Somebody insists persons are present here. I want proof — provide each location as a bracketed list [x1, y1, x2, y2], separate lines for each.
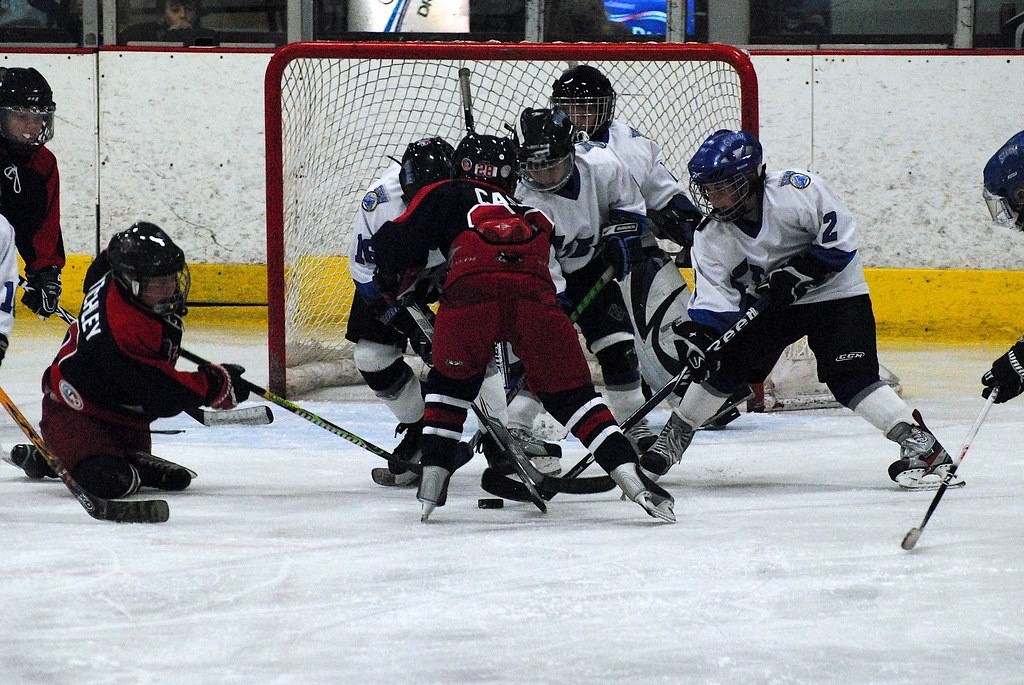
[0, 222, 252, 500]
[635, 129, 966, 490]
[0, 67, 66, 318]
[980, 131, 1024, 404]
[0, 0, 84, 44]
[117, 0, 219, 45]
[0, 214, 19, 367]
[346, 64, 739, 523]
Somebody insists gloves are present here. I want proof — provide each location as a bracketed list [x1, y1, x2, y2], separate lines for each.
[372, 260, 423, 307]
[0, 333, 9, 366]
[981, 341, 1024, 404]
[591, 206, 649, 282]
[21, 265, 62, 318]
[652, 193, 703, 245]
[555, 290, 574, 317]
[671, 320, 722, 384]
[738, 262, 816, 317]
[198, 361, 250, 410]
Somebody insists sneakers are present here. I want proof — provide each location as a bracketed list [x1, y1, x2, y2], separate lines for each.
[506, 428, 562, 478]
[388, 414, 426, 486]
[130, 449, 197, 493]
[639, 407, 700, 482]
[416, 442, 474, 521]
[0, 444, 60, 480]
[593, 431, 677, 523]
[697, 407, 741, 430]
[701, 383, 755, 427]
[624, 419, 659, 461]
[884, 408, 967, 492]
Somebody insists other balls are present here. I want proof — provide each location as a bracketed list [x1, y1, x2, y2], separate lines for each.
[478, 498, 504, 509]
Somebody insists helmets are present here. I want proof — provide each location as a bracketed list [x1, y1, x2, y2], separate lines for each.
[399, 137, 455, 205]
[451, 131, 520, 197]
[518, 107, 578, 194]
[983, 130, 1024, 213]
[552, 65, 614, 142]
[0, 66, 56, 155]
[687, 129, 762, 224]
[108, 220, 191, 318]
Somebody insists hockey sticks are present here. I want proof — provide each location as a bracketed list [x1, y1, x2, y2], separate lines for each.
[0, 386, 170, 521]
[466, 399, 549, 513]
[901, 386, 1000, 549]
[405, 300, 616, 493]
[17, 274, 273, 427]
[175, 342, 475, 476]
[468, 267, 614, 447]
[458, 67, 511, 388]
[480, 294, 772, 503]
